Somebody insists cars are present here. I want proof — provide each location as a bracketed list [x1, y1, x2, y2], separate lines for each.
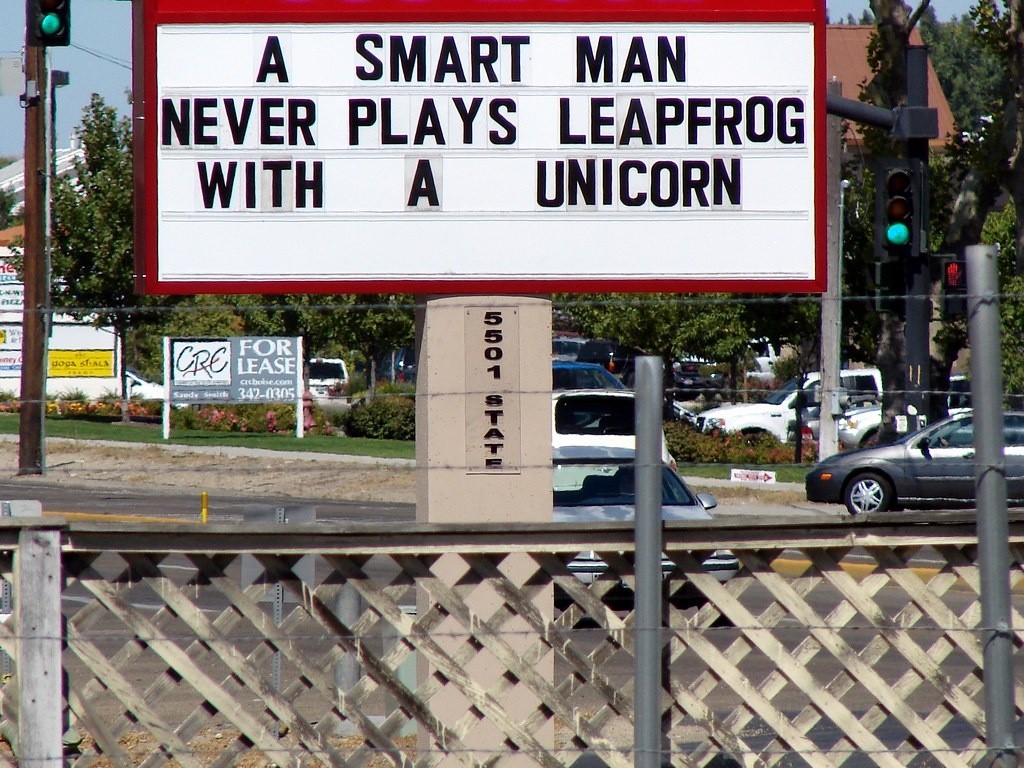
[550, 388, 679, 472]
[544, 445, 741, 594]
[671, 360, 724, 398]
[805, 407, 1024, 515]
[552, 356, 633, 392]
[123, 368, 190, 409]
[694, 369, 1009, 450]
[553, 336, 642, 382]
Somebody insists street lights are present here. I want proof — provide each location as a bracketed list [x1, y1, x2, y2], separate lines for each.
[50, 68, 71, 180]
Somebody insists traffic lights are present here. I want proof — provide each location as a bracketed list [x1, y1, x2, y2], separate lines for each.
[874, 161, 919, 259]
[941, 259, 967, 293]
[27, 0, 72, 47]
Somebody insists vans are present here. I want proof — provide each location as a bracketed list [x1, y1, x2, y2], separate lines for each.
[381, 346, 417, 384]
[306, 359, 352, 408]
